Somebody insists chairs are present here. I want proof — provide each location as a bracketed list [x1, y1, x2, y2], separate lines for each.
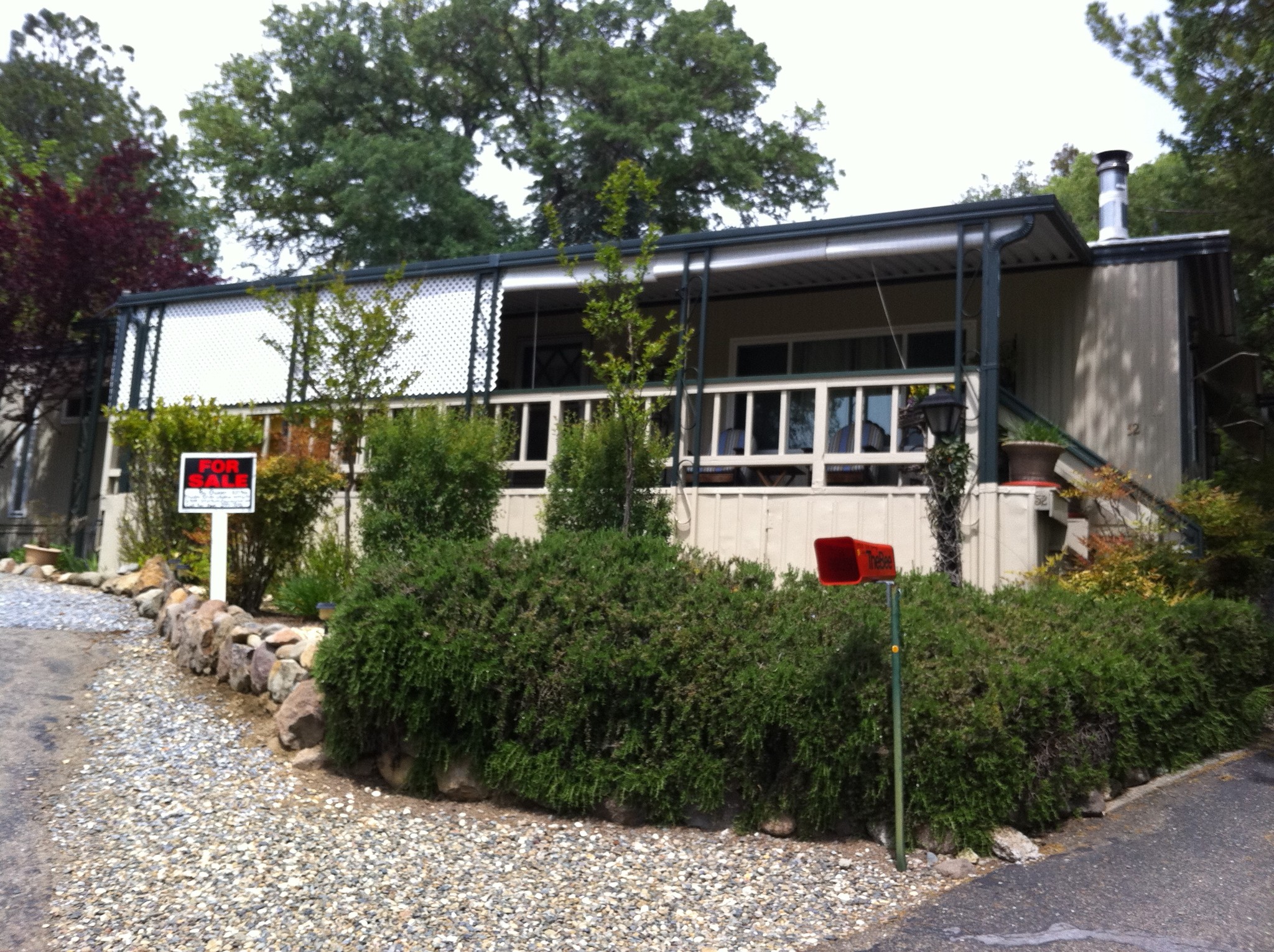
[800, 420, 890, 486]
[685, 428, 757, 487]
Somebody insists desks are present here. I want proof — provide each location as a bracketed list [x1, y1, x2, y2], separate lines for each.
[750, 462, 806, 487]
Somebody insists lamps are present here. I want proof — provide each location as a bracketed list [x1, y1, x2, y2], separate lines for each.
[919, 385, 965, 439]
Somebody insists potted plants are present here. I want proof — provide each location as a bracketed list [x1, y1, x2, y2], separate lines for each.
[22, 536, 63, 567]
[998, 414, 1070, 480]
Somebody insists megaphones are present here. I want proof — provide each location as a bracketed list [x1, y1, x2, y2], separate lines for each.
[813, 534, 896, 585]
